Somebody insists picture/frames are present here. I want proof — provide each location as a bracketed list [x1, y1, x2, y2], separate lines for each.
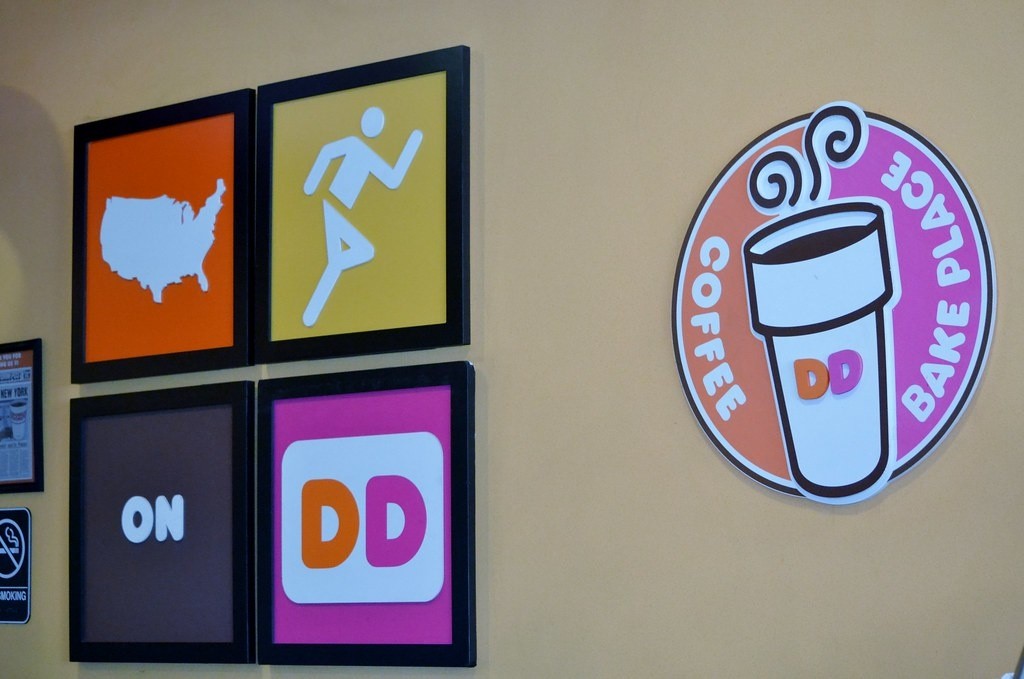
[70, 88, 251, 383]
[257, 361, 479, 667]
[69, 381, 252, 663]
[0, 338, 45, 493]
[254, 45, 470, 364]
